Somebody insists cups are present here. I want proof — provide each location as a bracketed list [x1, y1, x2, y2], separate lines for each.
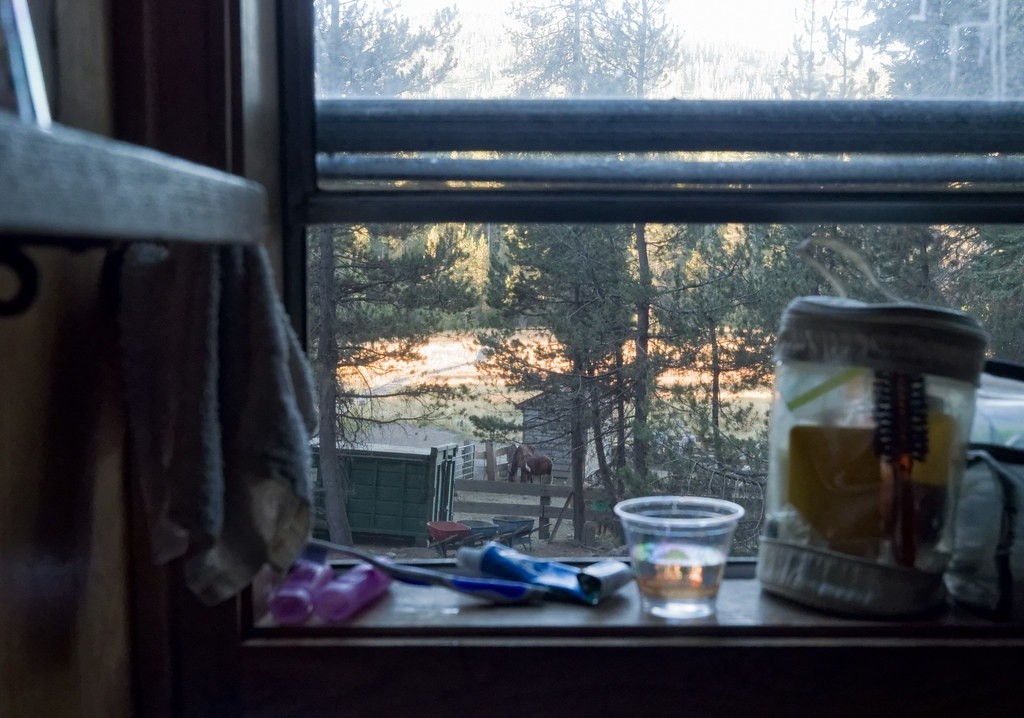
[613, 497, 747, 619]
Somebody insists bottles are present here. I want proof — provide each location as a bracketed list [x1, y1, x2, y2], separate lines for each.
[313, 554, 394, 622]
[269, 546, 334, 622]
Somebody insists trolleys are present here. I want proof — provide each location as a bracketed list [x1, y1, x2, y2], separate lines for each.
[426, 515, 552, 559]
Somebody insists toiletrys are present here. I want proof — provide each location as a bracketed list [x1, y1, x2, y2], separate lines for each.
[453, 539, 634, 603]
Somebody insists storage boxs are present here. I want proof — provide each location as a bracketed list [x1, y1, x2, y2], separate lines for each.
[311, 440, 459, 538]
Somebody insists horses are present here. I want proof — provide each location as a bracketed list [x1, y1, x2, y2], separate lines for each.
[509, 444, 553, 483]
